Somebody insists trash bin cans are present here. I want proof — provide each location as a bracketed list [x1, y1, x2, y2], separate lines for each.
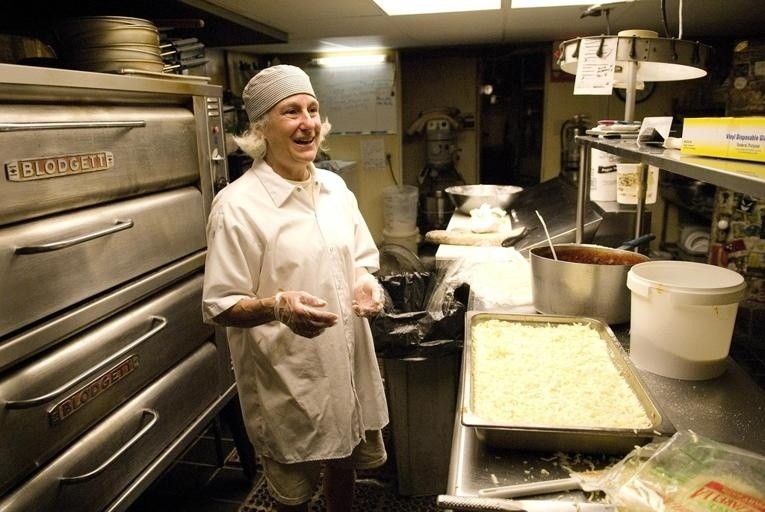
[371, 272, 470, 498]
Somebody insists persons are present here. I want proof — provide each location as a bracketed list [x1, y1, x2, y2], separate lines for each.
[202, 63, 391, 512]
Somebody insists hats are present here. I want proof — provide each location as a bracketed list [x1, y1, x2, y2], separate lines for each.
[243, 64, 316, 122]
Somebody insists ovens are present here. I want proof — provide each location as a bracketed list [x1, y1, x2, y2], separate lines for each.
[1, 66, 229, 512]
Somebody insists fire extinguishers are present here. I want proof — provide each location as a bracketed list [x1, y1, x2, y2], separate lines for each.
[561, 115, 588, 172]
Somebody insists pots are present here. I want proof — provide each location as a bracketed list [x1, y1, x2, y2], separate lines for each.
[74, 14, 210, 75]
[528, 230, 657, 326]
[661, 176, 716, 205]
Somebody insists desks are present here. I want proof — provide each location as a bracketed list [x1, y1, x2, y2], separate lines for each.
[436, 245, 765, 512]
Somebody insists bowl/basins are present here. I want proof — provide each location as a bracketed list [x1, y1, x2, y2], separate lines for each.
[443, 182, 524, 216]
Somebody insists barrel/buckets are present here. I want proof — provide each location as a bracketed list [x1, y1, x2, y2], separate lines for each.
[625, 260, 747, 381]
[382, 227, 419, 258]
[589, 148, 623, 202]
[616, 163, 659, 205]
